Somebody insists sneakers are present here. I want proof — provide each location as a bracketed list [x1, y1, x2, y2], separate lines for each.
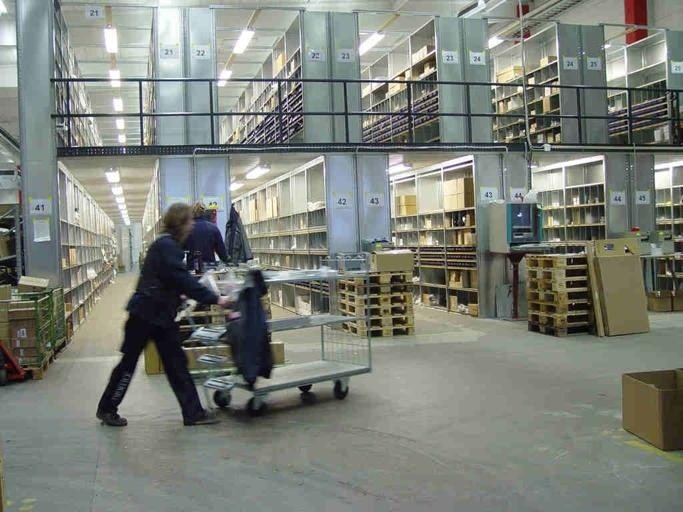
[94, 407, 128, 427]
[181, 410, 219, 426]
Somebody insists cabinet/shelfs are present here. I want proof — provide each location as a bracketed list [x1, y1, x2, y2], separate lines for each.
[0, 1, 681, 376]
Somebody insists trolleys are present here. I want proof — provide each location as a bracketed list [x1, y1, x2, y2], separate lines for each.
[179, 248, 377, 419]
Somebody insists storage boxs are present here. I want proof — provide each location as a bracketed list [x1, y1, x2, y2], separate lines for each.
[620, 365, 682, 454]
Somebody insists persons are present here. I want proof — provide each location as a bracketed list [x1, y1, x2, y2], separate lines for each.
[181, 202, 231, 268]
[96, 204, 235, 426]
